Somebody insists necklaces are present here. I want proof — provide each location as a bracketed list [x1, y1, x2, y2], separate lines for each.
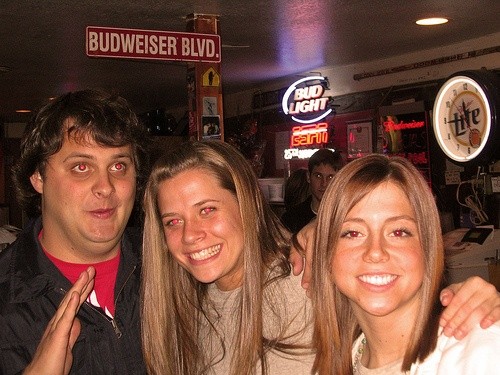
[348, 336, 368, 375]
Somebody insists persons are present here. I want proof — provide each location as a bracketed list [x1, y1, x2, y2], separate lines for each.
[0, 86, 321, 375]
[308, 152, 500, 375]
[282, 168, 312, 210]
[278, 148, 344, 233]
[135, 137, 499, 375]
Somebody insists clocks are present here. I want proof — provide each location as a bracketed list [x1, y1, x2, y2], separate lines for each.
[433, 70, 500, 167]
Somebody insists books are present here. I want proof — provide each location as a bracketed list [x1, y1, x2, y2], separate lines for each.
[462, 225, 494, 246]
